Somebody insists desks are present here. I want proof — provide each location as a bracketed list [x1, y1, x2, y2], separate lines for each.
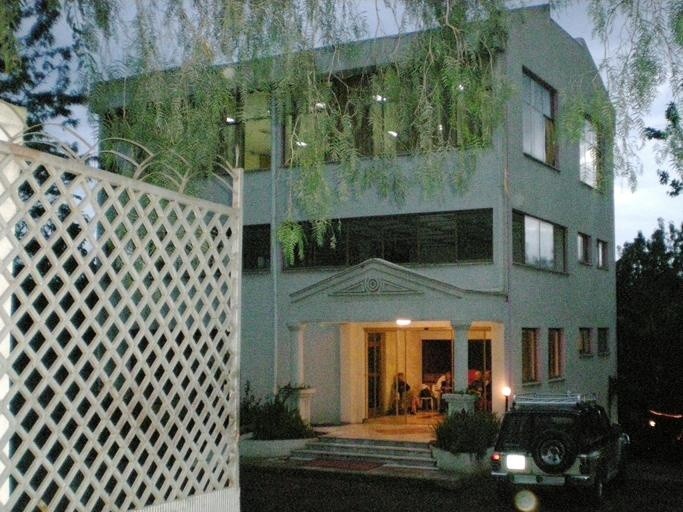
[421, 397, 433, 410]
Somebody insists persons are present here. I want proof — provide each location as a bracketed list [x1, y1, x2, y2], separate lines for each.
[437, 372, 452, 414]
[393, 373, 419, 416]
[485, 368, 490, 403]
[469, 368, 485, 409]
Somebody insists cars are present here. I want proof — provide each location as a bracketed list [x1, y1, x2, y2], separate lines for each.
[636, 398, 683, 458]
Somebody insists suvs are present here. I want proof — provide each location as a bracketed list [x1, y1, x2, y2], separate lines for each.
[490, 392, 632, 506]
